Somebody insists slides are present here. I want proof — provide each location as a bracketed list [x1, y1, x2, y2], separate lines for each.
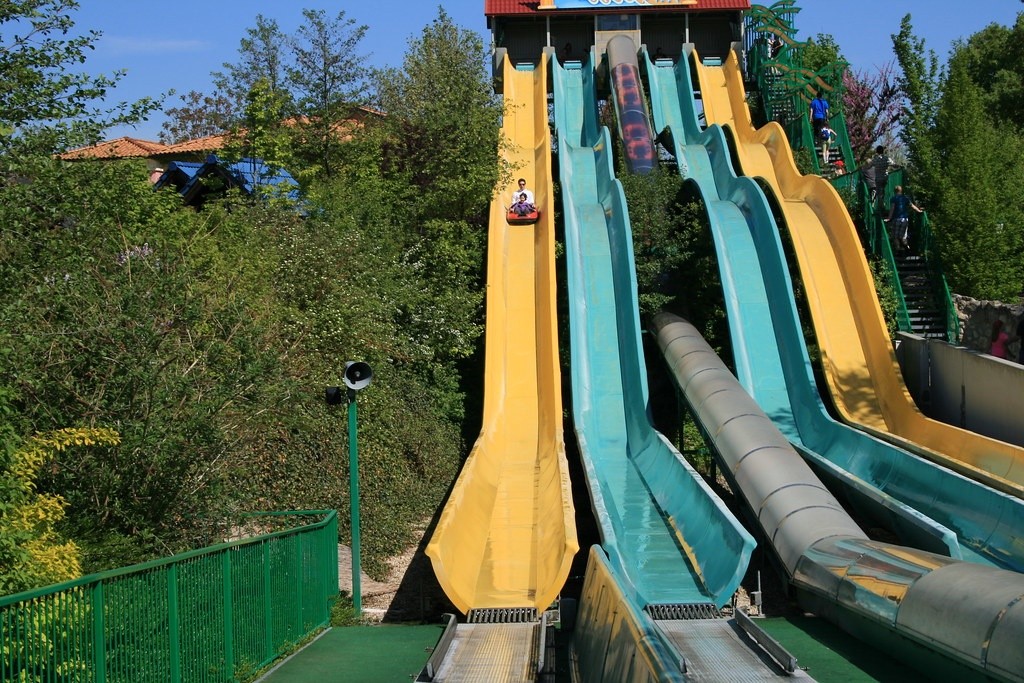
[424, 44, 1022, 614]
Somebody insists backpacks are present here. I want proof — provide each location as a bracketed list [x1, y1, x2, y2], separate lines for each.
[821, 128, 831, 141]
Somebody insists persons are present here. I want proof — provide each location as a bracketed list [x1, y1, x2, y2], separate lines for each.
[767, 34, 782, 74]
[985, 320, 1016, 360]
[509, 179, 536, 217]
[810, 92, 829, 147]
[814, 161, 846, 178]
[650, 48, 665, 64]
[862, 146, 889, 215]
[883, 185, 923, 250]
[1016, 312, 1024, 365]
[820, 123, 837, 165]
